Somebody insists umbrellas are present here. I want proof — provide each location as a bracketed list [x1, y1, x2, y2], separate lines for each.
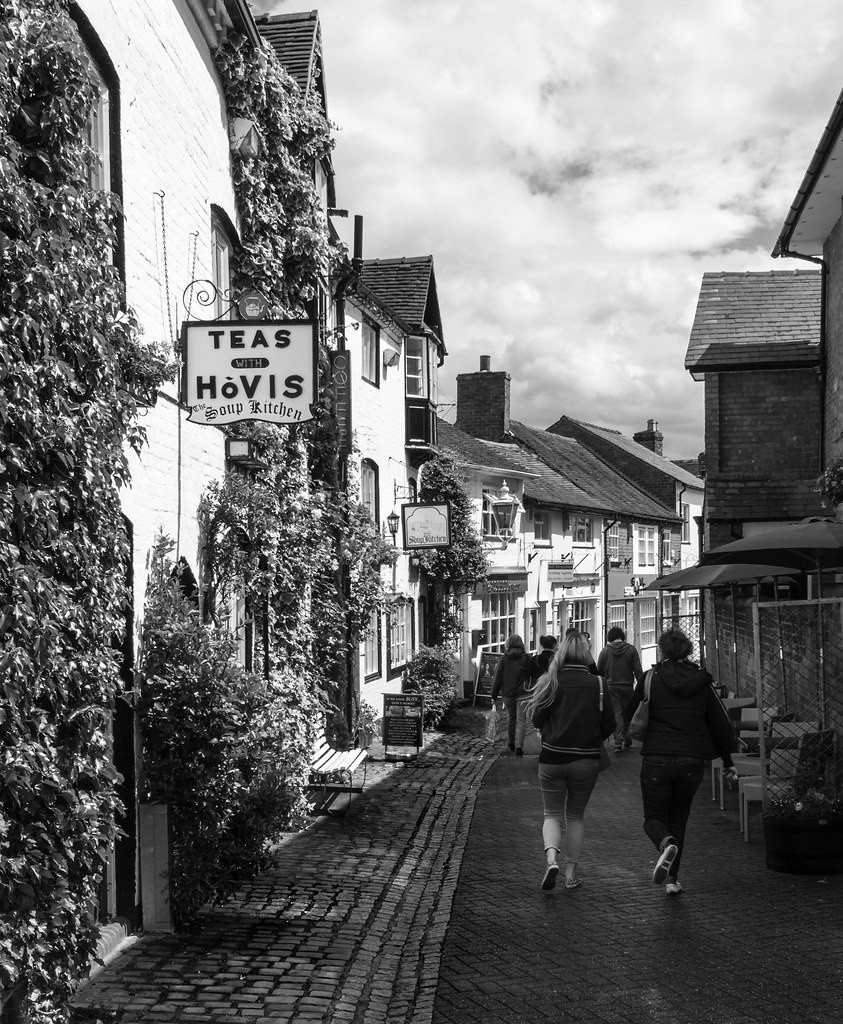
[635, 522, 843, 730]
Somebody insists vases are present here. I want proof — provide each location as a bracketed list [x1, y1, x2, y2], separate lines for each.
[833, 503, 843, 520]
[760, 812, 842, 875]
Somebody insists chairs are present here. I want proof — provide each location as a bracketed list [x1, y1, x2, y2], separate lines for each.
[737, 722, 818, 832]
[710, 707, 778, 802]
[743, 729, 836, 841]
[719, 712, 794, 809]
[721, 696, 755, 721]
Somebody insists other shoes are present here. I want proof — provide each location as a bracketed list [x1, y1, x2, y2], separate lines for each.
[565, 873, 582, 889]
[614, 747, 621, 752]
[516, 748, 523, 755]
[653, 845, 678, 884]
[508, 743, 515, 751]
[665, 881, 681, 894]
[541, 860, 559, 890]
[624, 742, 632, 748]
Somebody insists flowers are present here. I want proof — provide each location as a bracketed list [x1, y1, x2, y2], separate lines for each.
[816, 456, 843, 509]
[763, 776, 842, 817]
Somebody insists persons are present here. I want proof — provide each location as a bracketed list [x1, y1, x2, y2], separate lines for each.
[625, 630, 738, 895]
[531, 627, 643, 753]
[491, 635, 530, 754]
[521, 633, 617, 889]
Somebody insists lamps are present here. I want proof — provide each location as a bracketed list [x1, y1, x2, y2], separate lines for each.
[386, 510, 400, 534]
[480, 478, 520, 551]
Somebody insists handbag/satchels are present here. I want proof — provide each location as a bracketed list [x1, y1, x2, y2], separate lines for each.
[599, 740, 610, 772]
[628, 666, 655, 742]
[485, 704, 500, 743]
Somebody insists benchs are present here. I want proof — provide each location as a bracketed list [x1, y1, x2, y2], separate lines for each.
[303, 711, 367, 815]
